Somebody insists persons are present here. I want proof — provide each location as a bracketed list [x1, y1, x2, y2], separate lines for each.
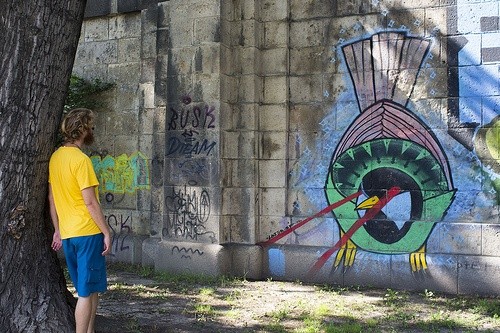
[48, 108, 113, 333]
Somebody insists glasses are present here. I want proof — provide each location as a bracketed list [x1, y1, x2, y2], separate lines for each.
[87, 124, 97, 131]
